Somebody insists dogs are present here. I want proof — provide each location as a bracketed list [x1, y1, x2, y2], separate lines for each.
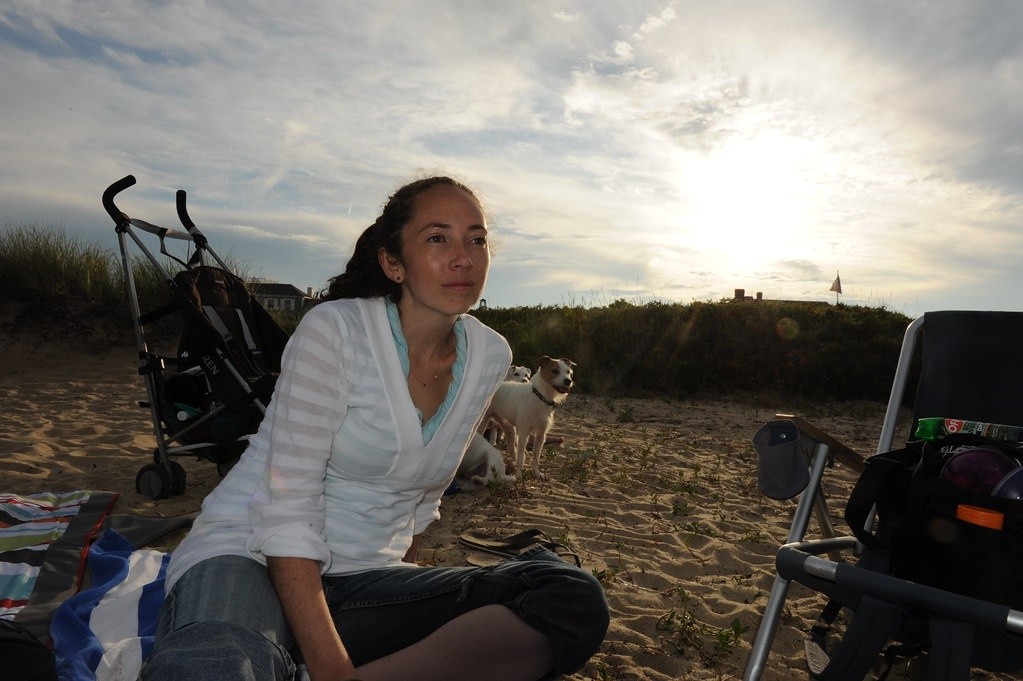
[476, 356, 578, 480]
[486, 364, 531, 445]
[453, 430, 516, 486]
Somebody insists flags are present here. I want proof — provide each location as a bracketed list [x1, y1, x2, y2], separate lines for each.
[829, 276, 843, 294]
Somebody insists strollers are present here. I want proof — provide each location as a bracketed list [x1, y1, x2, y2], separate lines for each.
[103, 175, 296, 498]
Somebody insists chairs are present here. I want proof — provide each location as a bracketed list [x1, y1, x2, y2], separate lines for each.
[730, 309, 1023, 681]
[175, 257, 291, 446]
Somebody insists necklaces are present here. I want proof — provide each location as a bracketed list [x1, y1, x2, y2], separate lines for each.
[406, 331, 456, 388]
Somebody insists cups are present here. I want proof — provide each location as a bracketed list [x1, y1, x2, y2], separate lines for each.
[956, 504, 1005, 530]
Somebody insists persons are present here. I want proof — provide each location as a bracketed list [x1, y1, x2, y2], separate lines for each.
[124, 176, 611, 681]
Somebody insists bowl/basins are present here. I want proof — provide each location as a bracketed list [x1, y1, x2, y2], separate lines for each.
[941, 445, 1017, 496]
[991, 466, 1023, 501]
